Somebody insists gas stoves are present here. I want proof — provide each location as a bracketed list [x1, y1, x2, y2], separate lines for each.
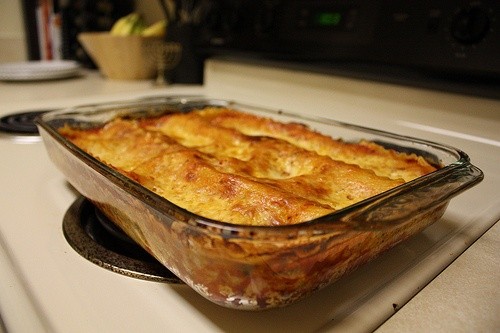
[1, 84, 500, 333]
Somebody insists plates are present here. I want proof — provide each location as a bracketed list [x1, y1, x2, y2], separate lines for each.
[0, 61, 81, 80]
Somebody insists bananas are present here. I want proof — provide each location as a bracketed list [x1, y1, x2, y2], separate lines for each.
[110, 11, 167, 35]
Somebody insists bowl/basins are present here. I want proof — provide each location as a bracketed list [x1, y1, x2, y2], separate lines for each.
[79, 32, 165, 80]
[34, 95, 485, 311]
